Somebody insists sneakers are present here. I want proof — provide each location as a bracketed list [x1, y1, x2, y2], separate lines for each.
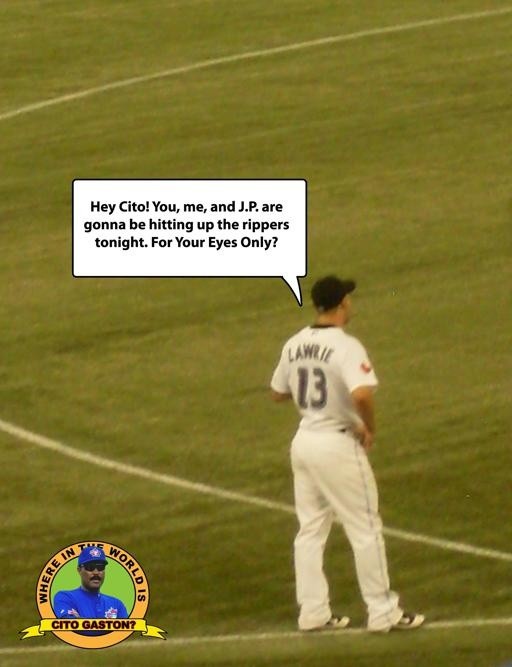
[299, 616, 349, 632]
[374, 612, 425, 632]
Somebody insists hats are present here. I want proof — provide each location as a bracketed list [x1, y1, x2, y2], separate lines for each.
[311, 278, 355, 302]
[78, 547, 108, 567]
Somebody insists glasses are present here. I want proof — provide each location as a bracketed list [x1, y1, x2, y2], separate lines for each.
[79, 564, 106, 571]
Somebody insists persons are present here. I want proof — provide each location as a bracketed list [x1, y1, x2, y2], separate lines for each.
[54, 547, 129, 636]
[270, 275, 426, 633]
